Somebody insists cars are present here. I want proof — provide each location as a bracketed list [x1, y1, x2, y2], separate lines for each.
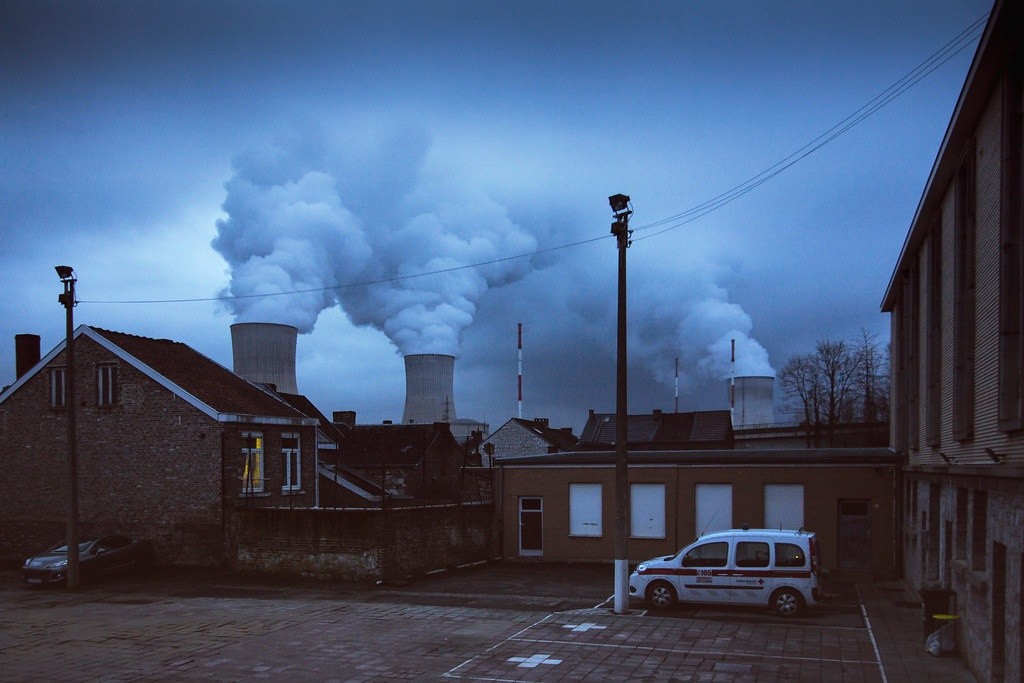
[629, 529, 824, 617]
[22, 533, 154, 584]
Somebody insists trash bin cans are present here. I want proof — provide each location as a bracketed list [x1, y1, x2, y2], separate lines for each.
[915, 577, 958, 645]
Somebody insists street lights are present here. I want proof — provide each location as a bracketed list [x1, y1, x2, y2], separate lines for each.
[609, 195, 632, 614]
[56, 266, 80, 588]
